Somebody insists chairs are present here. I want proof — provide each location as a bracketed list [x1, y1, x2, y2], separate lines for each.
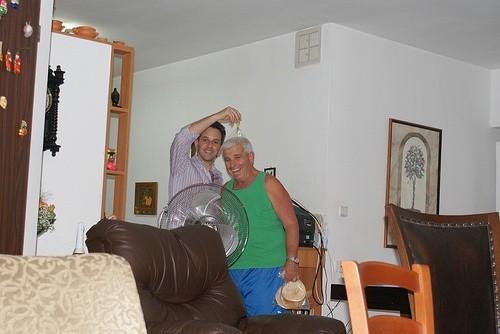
[385, 204, 500, 334]
[342, 259, 435, 334]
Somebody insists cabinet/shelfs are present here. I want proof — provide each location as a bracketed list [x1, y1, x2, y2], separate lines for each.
[101, 45, 134, 222]
[331, 284, 412, 319]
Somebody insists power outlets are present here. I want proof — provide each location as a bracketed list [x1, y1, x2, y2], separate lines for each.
[339, 206, 348, 217]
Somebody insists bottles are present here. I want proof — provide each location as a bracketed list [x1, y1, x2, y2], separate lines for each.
[72, 221, 85, 254]
[111, 88, 119, 106]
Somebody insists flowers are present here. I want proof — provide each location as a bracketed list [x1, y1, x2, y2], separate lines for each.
[37, 198, 57, 238]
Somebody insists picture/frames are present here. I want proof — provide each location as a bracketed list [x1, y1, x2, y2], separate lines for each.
[384, 118, 442, 248]
[264, 168, 276, 177]
[134, 182, 158, 215]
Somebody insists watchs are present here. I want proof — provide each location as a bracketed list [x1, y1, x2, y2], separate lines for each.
[288, 256, 300, 264]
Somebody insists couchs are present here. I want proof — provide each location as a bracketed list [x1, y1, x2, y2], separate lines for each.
[0, 254, 148, 334]
[85, 217, 346, 334]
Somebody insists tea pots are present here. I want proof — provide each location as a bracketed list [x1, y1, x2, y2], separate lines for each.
[52, 20, 65, 32]
[72, 26, 98, 39]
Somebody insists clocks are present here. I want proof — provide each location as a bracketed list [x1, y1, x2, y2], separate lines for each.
[42, 63, 66, 156]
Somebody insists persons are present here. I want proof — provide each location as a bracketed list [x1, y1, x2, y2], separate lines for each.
[167, 107, 243, 229]
[220, 135, 302, 316]
